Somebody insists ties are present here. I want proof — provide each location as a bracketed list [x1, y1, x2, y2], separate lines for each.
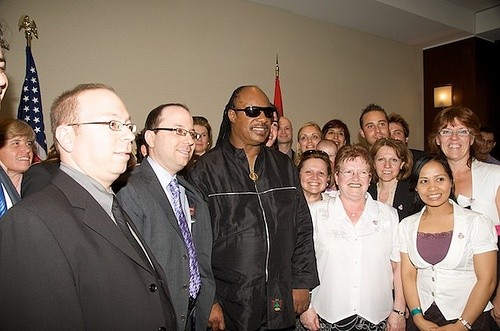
[170, 179, 201, 299]
[0, 184, 7, 217]
[112, 193, 178, 330]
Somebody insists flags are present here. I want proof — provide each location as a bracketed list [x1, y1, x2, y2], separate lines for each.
[17, 46, 47, 165]
[273, 79, 283, 117]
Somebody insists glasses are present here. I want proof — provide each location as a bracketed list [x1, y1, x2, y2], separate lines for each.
[301, 149, 328, 157]
[341, 168, 369, 178]
[439, 129, 469, 135]
[233, 106, 275, 118]
[151, 129, 198, 138]
[70, 120, 137, 131]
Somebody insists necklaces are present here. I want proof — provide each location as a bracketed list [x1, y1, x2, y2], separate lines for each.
[344, 207, 364, 217]
[451, 169, 470, 182]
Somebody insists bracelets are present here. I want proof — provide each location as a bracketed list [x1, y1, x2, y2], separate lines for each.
[457, 316, 472, 331]
[411, 307, 422, 317]
[393, 309, 407, 317]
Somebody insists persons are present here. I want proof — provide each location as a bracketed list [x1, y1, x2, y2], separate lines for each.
[359, 102, 390, 151]
[426, 105, 500, 328]
[116, 103, 217, 330]
[134, 128, 148, 165]
[316, 138, 339, 191]
[184, 85, 320, 331]
[387, 112, 426, 173]
[322, 119, 350, 153]
[1, 118, 37, 198]
[296, 151, 332, 203]
[185, 116, 213, 172]
[266, 103, 280, 148]
[0, 17, 21, 219]
[293, 122, 325, 167]
[471, 127, 500, 166]
[398, 153, 499, 331]
[296, 143, 408, 331]
[273, 115, 296, 163]
[367, 137, 421, 223]
[1, 83, 178, 331]
